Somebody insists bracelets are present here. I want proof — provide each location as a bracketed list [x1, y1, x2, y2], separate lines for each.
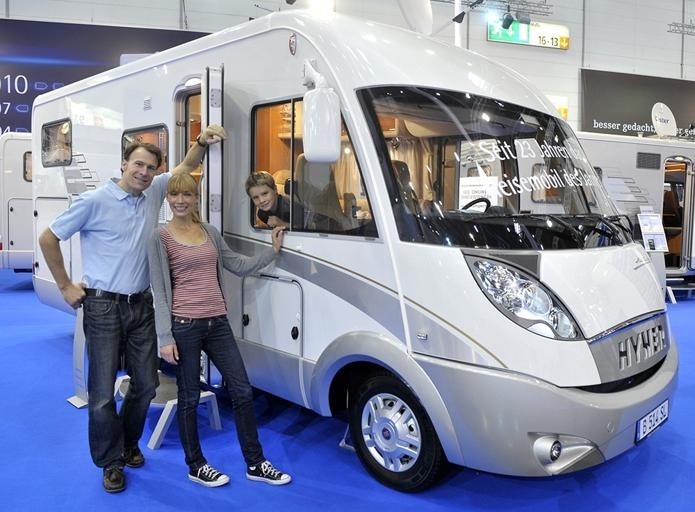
[196, 135, 205, 147]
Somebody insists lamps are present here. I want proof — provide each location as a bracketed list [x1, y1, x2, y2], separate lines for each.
[500, 4, 513, 30]
[515, 10, 531, 25]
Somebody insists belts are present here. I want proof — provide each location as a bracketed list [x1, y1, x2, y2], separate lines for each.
[82, 287, 143, 305]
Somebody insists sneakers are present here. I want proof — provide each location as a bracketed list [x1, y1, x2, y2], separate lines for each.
[188, 463, 230, 488]
[245, 459, 292, 486]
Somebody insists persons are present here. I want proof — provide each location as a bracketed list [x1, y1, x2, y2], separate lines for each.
[39, 125, 229, 494]
[244, 170, 341, 231]
[146, 173, 293, 486]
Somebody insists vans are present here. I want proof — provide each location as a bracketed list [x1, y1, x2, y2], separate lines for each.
[0, 132, 35, 271]
[455, 132, 695, 291]
[32, 9, 680, 493]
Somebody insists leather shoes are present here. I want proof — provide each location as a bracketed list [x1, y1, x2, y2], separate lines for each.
[120, 440, 145, 468]
[102, 463, 127, 493]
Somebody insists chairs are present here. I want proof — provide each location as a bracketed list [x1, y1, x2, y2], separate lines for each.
[271, 169, 300, 200]
[289, 154, 361, 235]
[663, 190, 683, 237]
[393, 159, 422, 216]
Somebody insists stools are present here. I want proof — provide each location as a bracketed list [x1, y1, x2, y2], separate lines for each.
[113, 368, 223, 450]
